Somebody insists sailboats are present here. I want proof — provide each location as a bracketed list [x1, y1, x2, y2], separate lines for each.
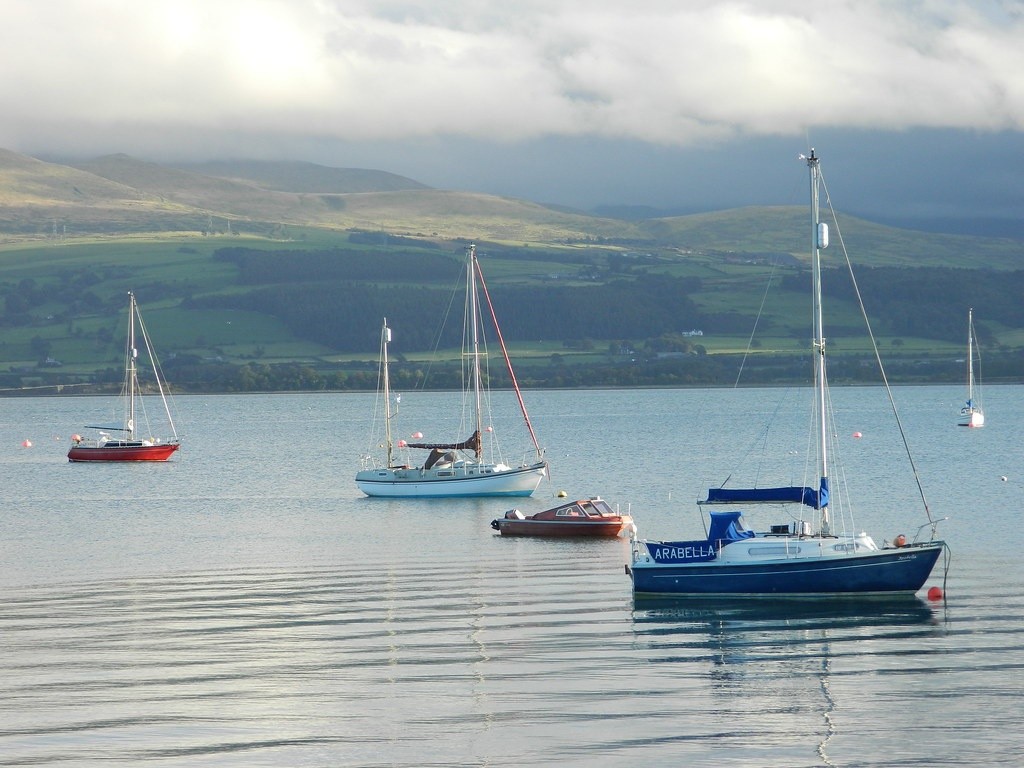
[622, 147, 956, 604]
[66, 290, 183, 462]
[958, 310, 987, 426]
[355, 244, 552, 499]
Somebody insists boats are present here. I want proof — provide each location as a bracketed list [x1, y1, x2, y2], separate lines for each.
[489, 496, 635, 539]
